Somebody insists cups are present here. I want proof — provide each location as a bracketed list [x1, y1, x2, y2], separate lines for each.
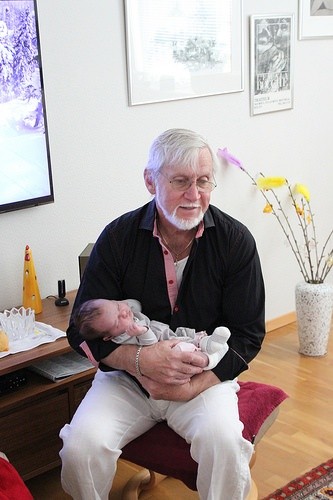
[0, 306, 36, 343]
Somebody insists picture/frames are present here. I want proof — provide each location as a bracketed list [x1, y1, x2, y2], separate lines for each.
[297, 0, 333, 40]
[122, 0, 244, 107]
[250, 12, 295, 117]
[0, 0, 55, 215]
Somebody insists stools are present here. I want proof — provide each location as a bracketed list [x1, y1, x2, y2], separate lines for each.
[118, 378, 290, 500]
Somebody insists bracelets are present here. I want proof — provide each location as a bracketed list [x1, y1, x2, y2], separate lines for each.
[136, 346, 143, 376]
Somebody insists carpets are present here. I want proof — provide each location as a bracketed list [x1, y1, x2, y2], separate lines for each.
[261, 457, 333, 500]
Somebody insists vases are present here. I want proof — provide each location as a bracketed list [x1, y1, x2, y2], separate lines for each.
[295, 279, 333, 358]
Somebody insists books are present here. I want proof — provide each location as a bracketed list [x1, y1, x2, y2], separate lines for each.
[29, 350, 96, 382]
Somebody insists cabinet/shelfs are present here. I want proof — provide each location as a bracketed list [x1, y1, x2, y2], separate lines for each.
[0, 288, 98, 483]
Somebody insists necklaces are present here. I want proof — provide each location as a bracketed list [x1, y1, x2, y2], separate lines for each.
[156, 215, 198, 265]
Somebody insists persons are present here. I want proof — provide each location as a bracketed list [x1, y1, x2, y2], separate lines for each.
[73, 299, 231, 370]
[59, 128, 266, 500]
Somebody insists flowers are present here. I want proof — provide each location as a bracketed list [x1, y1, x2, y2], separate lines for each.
[218, 148, 333, 285]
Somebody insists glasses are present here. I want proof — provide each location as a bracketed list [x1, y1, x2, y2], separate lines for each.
[156, 169, 217, 193]
